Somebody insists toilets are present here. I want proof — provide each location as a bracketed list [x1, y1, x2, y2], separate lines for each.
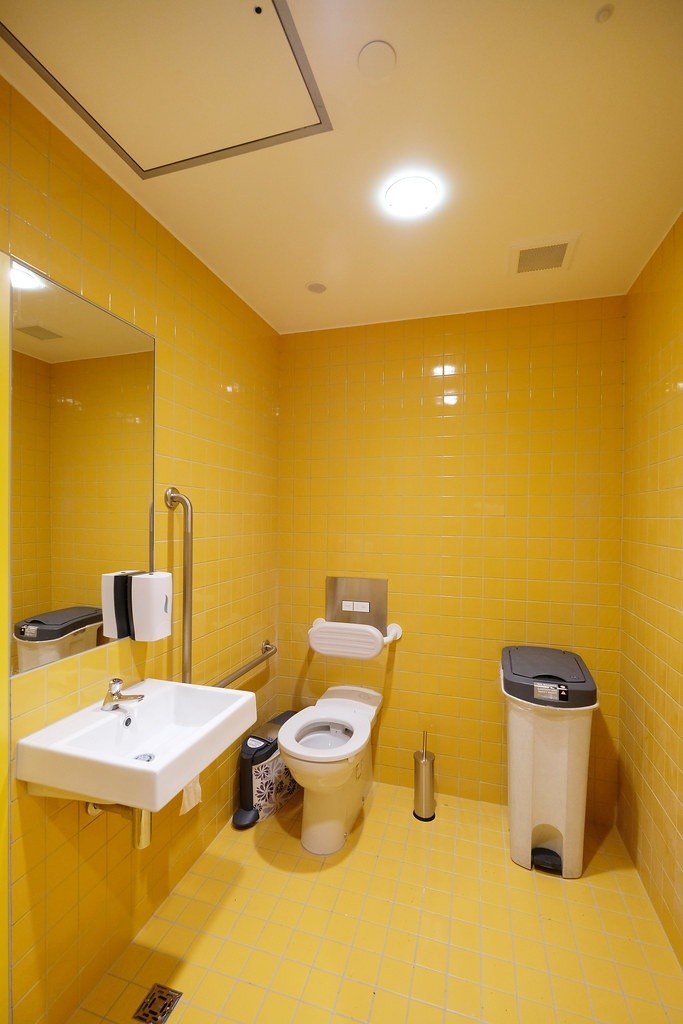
[276, 685, 383, 855]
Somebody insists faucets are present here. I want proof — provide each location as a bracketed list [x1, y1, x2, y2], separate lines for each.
[100, 678, 145, 711]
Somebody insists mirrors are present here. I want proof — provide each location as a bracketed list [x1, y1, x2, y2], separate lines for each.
[1, 250, 156, 677]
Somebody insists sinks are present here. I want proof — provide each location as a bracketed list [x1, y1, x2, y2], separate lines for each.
[16, 677, 257, 812]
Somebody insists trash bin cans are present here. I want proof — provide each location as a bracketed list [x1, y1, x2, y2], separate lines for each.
[13, 605, 103, 671]
[498, 643, 602, 882]
